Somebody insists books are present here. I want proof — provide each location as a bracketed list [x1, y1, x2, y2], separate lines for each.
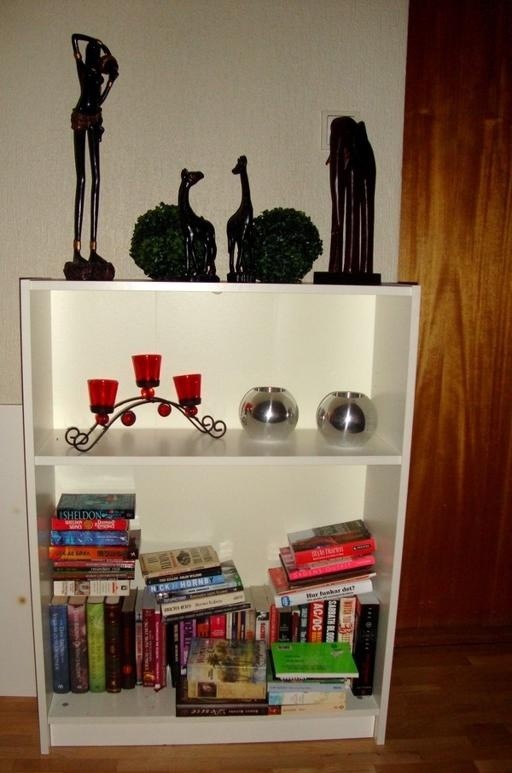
[46, 491, 383, 719]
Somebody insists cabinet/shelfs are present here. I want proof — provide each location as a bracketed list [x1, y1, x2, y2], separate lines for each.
[19, 278, 421, 755]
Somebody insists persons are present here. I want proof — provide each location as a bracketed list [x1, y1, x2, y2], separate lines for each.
[68, 30, 120, 270]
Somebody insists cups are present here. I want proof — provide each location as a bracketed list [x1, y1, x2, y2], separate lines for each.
[132, 355, 163, 386]
[172, 373, 203, 405]
[88, 377, 117, 414]
